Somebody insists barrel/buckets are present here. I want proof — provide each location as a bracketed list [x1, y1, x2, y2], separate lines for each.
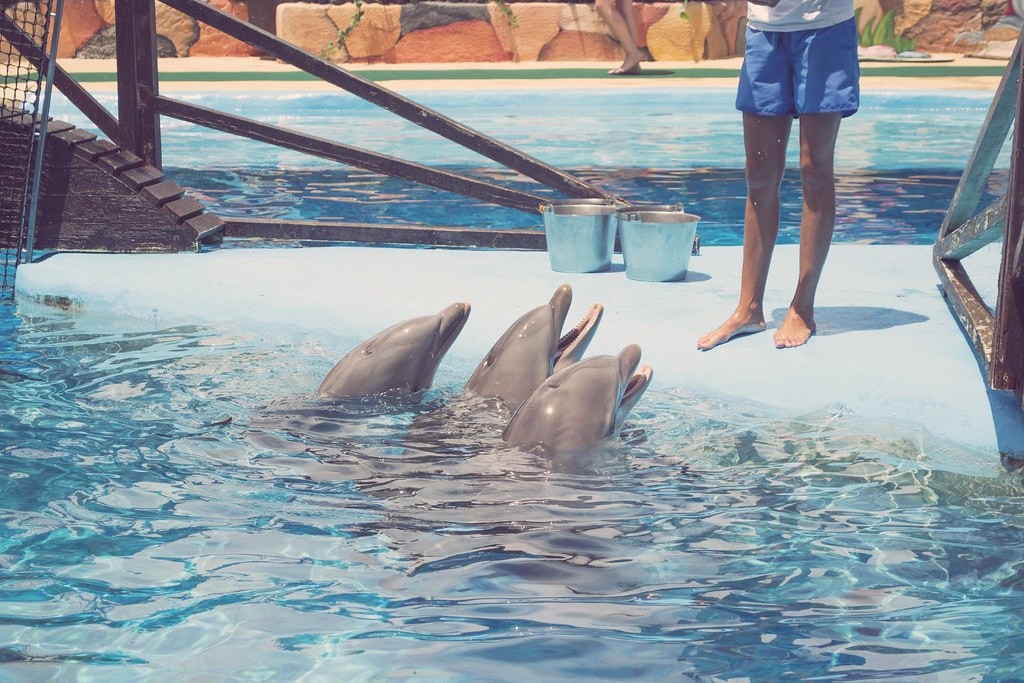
[614, 211, 701, 282]
[614, 204, 685, 215]
[542, 203, 618, 275]
[538, 197, 617, 214]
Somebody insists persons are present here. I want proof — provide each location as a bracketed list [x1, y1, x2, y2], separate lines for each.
[596, 0, 643, 75]
[697, 1, 862, 351]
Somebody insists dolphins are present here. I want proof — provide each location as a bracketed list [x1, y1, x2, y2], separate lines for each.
[462, 284, 604, 417]
[320, 304, 472, 406]
[504, 345, 654, 452]
